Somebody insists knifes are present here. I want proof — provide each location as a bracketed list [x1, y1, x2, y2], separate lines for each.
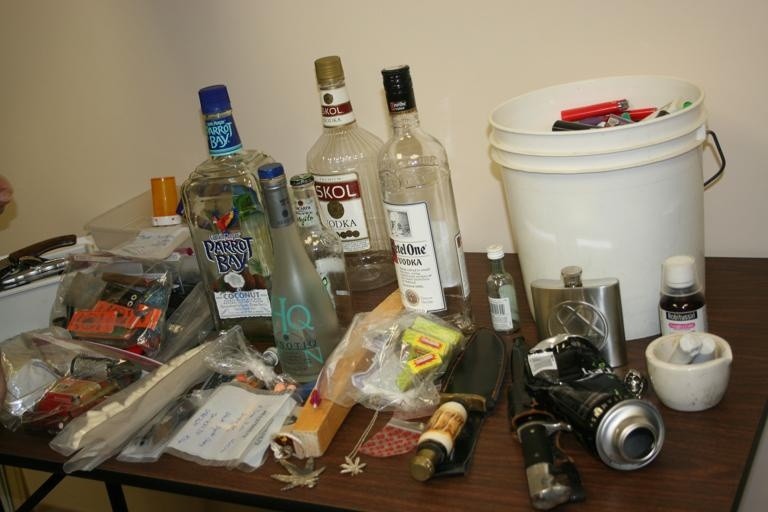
[507, 335, 573, 511]
[0, 233, 78, 273]
[409, 326, 506, 482]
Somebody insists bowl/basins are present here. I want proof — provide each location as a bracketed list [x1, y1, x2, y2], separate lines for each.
[645, 331, 733, 412]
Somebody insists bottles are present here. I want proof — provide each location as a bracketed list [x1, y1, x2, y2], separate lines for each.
[374, 64, 473, 325]
[528, 264, 627, 371]
[257, 162, 344, 417]
[288, 173, 357, 338]
[485, 242, 520, 336]
[68, 355, 131, 379]
[655, 256, 709, 336]
[304, 56, 397, 292]
[180, 83, 278, 348]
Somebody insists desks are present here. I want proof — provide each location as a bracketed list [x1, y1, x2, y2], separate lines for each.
[2, 217, 767, 512]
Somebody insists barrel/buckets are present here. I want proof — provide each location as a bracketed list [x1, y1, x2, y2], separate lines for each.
[488, 73, 726, 341]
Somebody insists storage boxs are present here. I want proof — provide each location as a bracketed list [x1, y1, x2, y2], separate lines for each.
[84, 184, 206, 289]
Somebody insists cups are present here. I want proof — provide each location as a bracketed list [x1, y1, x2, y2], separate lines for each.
[148, 176, 181, 227]
